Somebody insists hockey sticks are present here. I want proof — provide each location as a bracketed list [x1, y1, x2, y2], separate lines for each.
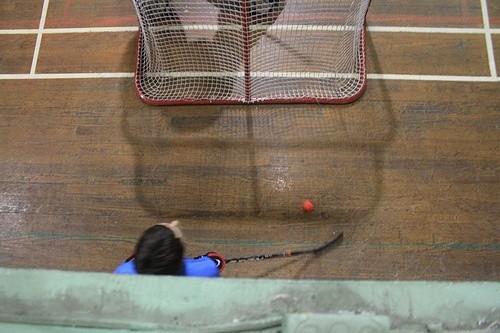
[225, 232, 345, 265]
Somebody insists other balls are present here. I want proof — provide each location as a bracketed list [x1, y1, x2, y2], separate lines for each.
[303, 199, 315, 211]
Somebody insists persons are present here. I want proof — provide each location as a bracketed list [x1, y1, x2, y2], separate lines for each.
[109, 219, 222, 279]
